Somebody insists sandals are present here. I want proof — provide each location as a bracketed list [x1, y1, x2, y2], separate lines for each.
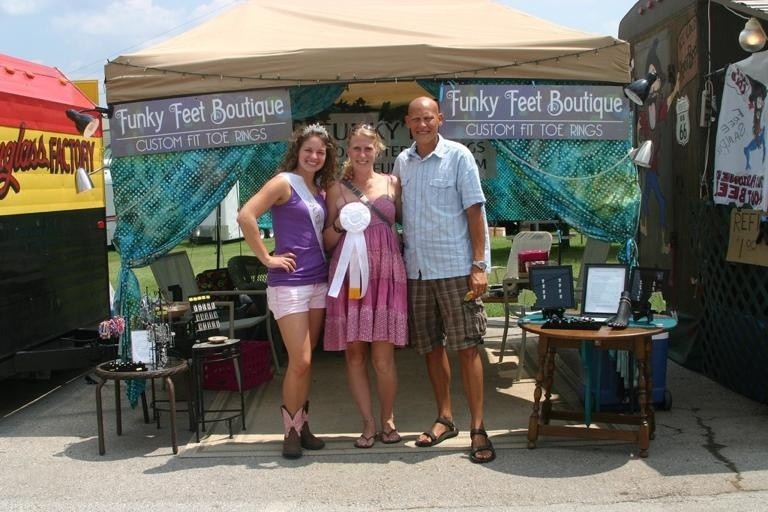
[470, 429, 496, 464]
[415, 418, 458, 448]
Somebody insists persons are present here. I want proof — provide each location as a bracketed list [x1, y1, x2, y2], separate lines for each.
[607, 290, 632, 329]
[389, 96, 497, 463]
[236, 120, 341, 460]
[323, 123, 403, 451]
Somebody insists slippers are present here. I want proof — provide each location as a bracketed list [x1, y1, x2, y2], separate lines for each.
[353, 434, 377, 449]
[381, 429, 402, 445]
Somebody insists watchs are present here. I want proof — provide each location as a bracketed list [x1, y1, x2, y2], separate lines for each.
[471, 259, 489, 272]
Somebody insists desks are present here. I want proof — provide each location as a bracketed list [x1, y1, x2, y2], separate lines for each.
[95, 354, 189, 457]
[191, 339, 247, 444]
[517, 313, 681, 458]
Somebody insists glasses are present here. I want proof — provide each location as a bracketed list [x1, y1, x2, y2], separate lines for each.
[348, 123, 378, 143]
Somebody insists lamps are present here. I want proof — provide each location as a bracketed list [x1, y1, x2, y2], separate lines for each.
[624, 73, 660, 108]
[64, 105, 115, 138]
[735, 17, 767, 53]
[633, 139, 661, 168]
[73, 163, 114, 194]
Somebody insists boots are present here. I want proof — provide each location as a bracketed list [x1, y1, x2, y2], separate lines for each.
[300, 400, 325, 451]
[280, 404, 305, 459]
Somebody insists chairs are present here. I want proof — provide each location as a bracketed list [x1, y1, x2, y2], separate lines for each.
[229, 255, 301, 368]
[481, 231, 556, 362]
[149, 251, 281, 394]
